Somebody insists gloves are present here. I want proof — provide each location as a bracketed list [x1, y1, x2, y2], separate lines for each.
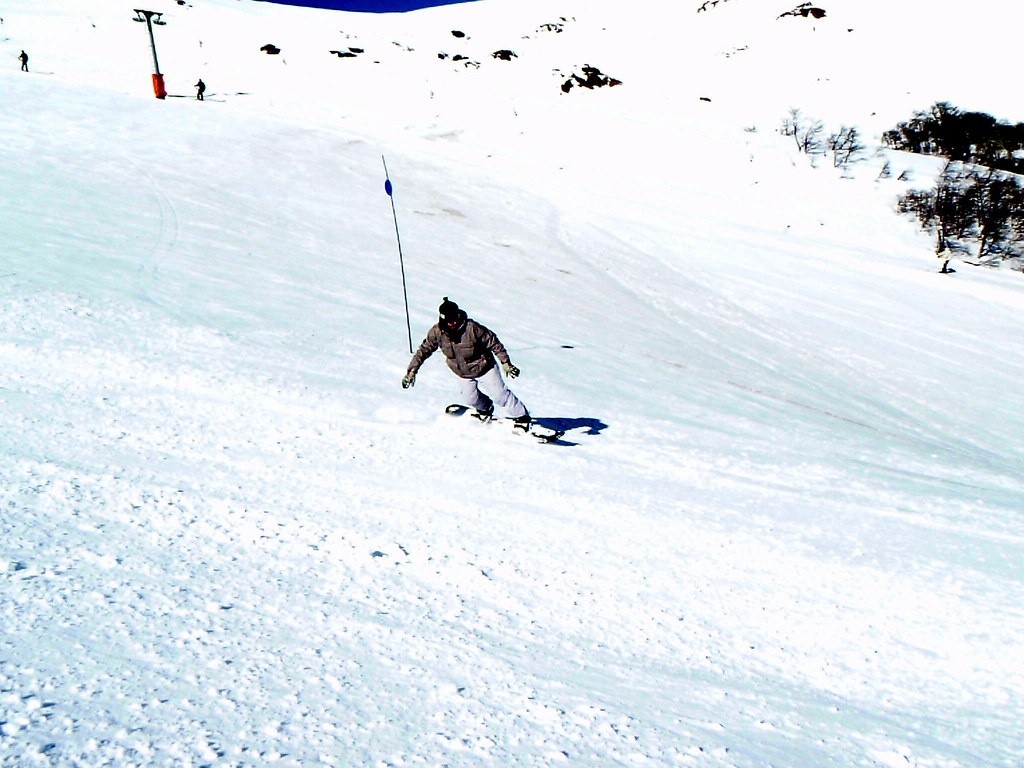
[503, 363, 520, 378]
[402, 373, 416, 389]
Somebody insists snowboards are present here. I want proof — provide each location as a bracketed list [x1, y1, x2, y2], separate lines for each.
[444, 403, 566, 445]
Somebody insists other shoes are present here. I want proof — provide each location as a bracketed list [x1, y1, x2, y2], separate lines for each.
[503, 406, 534, 435]
[470, 405, 496, 422]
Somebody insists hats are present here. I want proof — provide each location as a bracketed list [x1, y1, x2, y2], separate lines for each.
[438, 296, 458, 320]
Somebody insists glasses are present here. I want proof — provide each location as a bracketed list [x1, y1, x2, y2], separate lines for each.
[447, 321, 459, 324]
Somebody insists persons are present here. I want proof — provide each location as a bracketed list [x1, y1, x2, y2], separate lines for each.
[193, 77, 207, 99]
[403, 297, 534, 434]
[938, 245, 952, 273]
[18, 50, 29, 72]
